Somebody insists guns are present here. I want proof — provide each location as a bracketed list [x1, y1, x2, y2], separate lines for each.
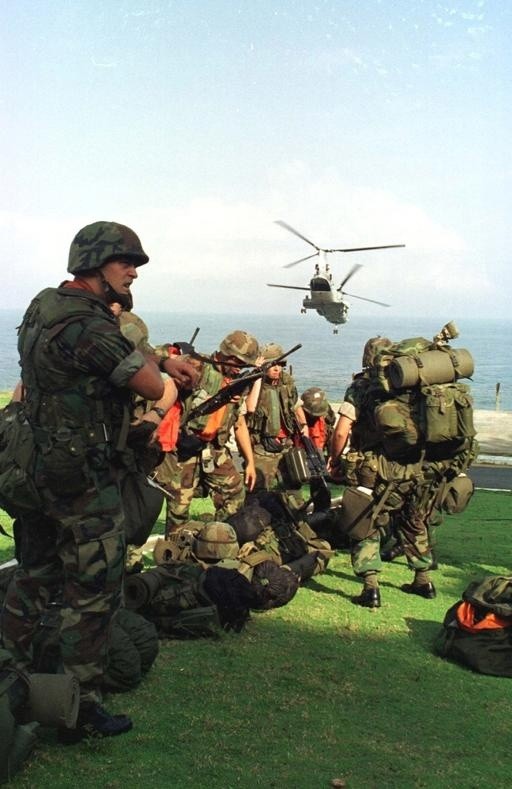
[187, 344, 302, 420]
[292, 418, 334, 497]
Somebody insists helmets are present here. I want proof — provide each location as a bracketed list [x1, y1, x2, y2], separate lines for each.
[67, 221, 149, 274]
[192, 521, 240, 559]
[219, 330, 259, 366]
[362, 338, 392, 367]
[301, 387, 328, 417]
[259, 343, 287, 367]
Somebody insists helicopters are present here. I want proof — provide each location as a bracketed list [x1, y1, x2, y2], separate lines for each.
[256, 219, 412, 335]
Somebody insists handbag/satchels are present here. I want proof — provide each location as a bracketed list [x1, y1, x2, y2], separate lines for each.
[0, 401, 44, 511]
[339, 487, 379, 541]
[441, 473, 475, 514]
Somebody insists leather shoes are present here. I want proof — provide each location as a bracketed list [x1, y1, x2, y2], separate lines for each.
[56, 698, 133, 746]
[352, 586, 381, 607]
[401, 580, 437, 599]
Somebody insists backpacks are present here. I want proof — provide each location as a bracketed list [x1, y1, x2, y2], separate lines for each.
[122, 561, 218, 641]
[1, 647, 41, 785]
[254, 519, 311, 567]
[372, 338, 476, 459]
[433, 576, 512, 678]
